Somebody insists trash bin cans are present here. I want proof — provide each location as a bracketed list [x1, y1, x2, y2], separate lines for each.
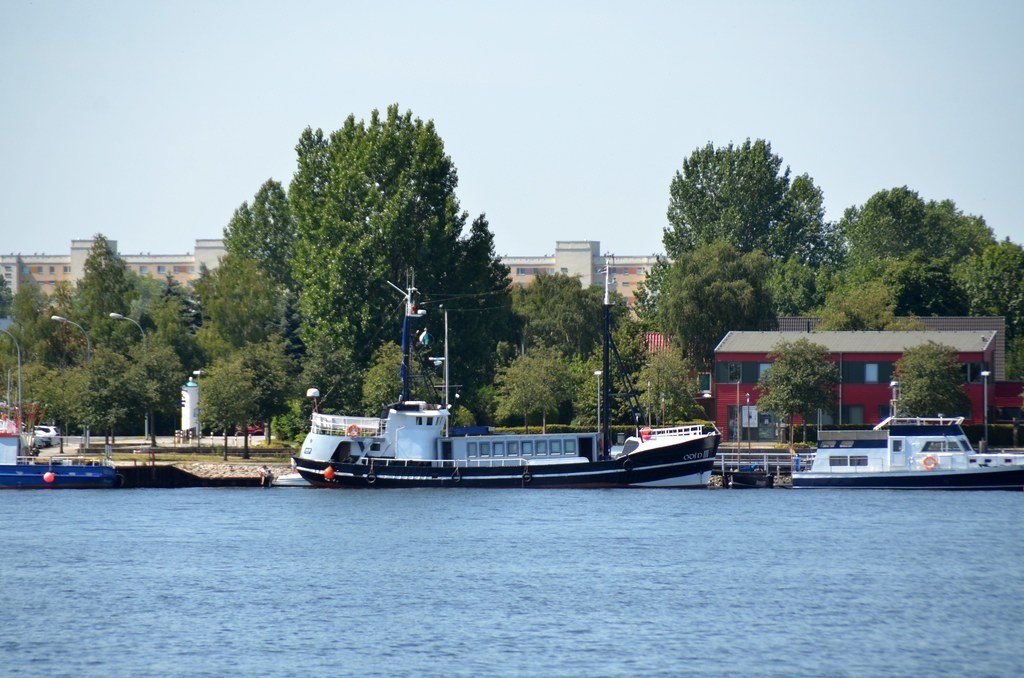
[617, 433, 625, 445]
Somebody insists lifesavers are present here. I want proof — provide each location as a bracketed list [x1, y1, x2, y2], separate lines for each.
[347, 424, 359, 437]
[624, 460, 634, 471]
[523, 472, 532, 482]
[451, 472, 461, 483]
[366, 472, 377, 484]
[923, 455, 937, 470]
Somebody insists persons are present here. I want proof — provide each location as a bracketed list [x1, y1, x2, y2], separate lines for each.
[258, 465, 275, 488]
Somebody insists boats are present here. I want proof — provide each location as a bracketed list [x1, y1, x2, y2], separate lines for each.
[792, 413, 1024, 489]
[0, 431, 120, 490]
[290, 259, 722, 492]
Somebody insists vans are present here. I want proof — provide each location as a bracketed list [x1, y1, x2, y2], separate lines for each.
[33, 426, 61, 447]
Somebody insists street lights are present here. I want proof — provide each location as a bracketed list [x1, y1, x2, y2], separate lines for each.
[109, 312, 150, 443]
[193, 369, 206, 446]
[594, 371, 601, 434]
[981, 371, 991, 440]
[50, 315, 91, 447]
